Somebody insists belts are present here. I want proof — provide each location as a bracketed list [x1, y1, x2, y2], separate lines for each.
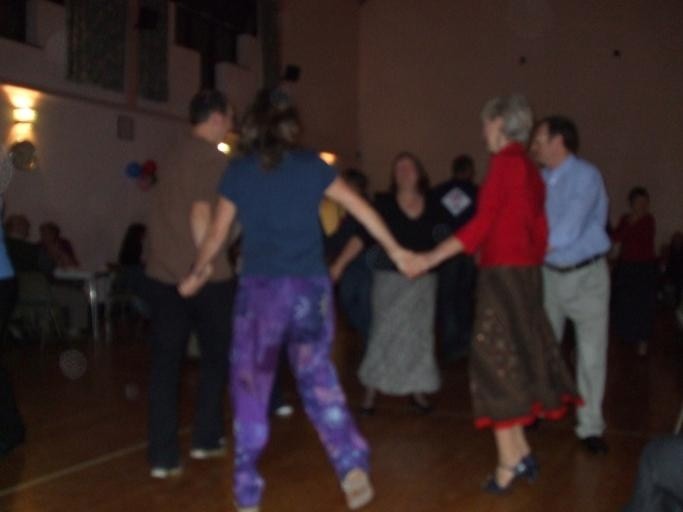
[543, 254, 601, 272]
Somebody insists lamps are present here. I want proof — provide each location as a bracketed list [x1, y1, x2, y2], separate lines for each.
[7, 139, 38, 173]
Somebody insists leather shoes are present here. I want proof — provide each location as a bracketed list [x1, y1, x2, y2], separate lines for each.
[582, 437, 607, 458]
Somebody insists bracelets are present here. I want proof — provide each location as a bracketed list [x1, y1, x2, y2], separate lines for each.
[188, 263, 206, 279]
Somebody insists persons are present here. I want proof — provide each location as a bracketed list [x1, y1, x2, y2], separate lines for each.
[179, 90, 421, 512]
[426, 153, 479, 240]
[143, 90, 233, 482]
[606, 186, 656, 362]
[0, 208, 151, 458]
[329, 150, 451, 416]
[633, 423, 681, 511]
[405, 91, 578, 497]
[527, 112, 613, 458]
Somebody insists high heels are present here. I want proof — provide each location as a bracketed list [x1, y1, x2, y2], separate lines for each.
[486, 454, 537, 493]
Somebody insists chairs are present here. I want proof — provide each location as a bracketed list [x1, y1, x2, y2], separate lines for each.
[88, 271, 129, 342]
[11, 279, 85, 357]
